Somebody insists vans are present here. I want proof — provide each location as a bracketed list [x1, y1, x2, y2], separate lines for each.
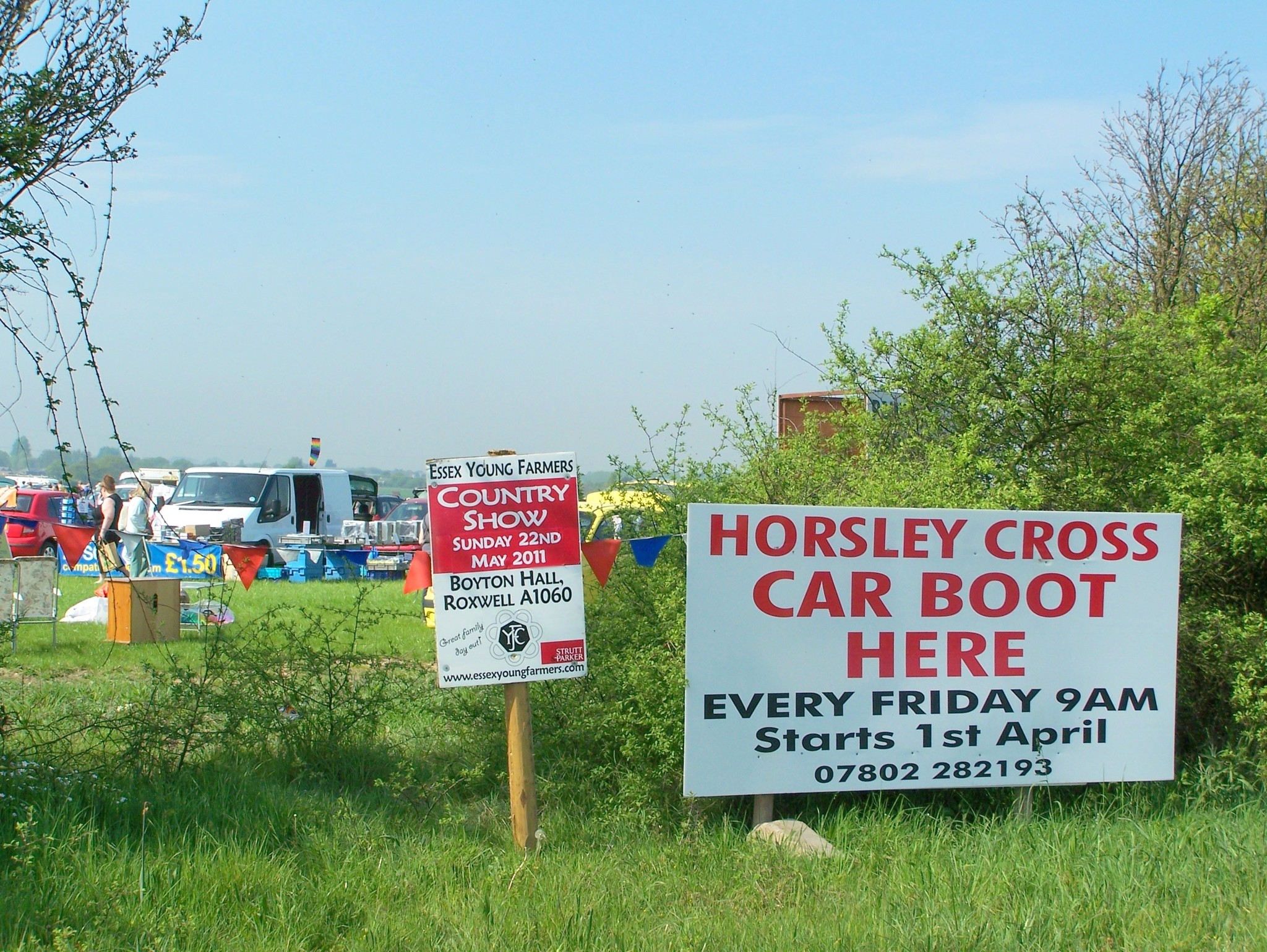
[147, 465, 354, 578]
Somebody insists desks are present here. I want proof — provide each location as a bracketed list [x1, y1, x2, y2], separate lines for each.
[180, 581, 228, 636]
[362, 543, 422, 551]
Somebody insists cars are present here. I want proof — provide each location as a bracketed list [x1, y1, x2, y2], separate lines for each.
[578, 476, 710, 614]
[348, 474, 429, 558]
[0, 468, 199, 566]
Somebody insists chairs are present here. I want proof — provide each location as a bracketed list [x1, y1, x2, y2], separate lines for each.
[14, 556, 62, 648]
[0, 557, 21, 650]
[354, 504, 372, 521]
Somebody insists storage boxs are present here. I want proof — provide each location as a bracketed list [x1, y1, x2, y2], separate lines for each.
[367, 563, 407, 571]
[284, 567, 324, 584]
[278, 535, 322, 544]
[324, 566, 362, 581]
[367, 570, 404, 581]
[152, 518, 245, 540]
[256, 566, 286, 579]
[325, 544, 360, 566]
[323, 536, 336, 544]
[284, 544, 325, 566]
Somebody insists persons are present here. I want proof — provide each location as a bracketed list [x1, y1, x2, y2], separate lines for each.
[92, 475, 129, 583]
[77, 480, 83, 490]
[26, 482, 32, 488]
[53, 486, 58, 491]
[21, 481, 28, 488]
[95, 481, 102, 491]
[58, 481, 65, 492]
[118, 481, 154, 578]
[83, 483, 91, 491]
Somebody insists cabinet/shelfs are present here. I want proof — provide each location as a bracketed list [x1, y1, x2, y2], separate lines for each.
[103, 577, 184, 644]
